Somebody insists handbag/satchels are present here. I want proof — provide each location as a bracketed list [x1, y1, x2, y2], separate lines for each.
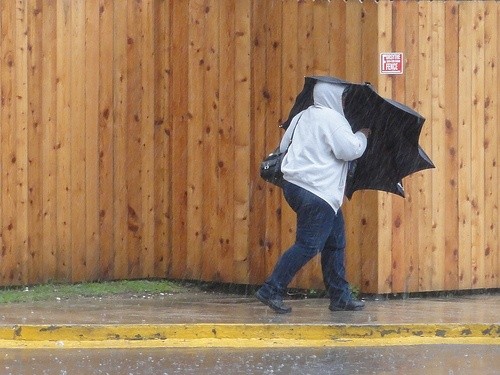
[259, 150, 289, 189]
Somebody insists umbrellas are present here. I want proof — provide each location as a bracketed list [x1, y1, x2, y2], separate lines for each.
[279, 77, 436, 201]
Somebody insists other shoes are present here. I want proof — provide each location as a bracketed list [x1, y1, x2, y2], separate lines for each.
[255, 290, 291, 313]
[328, 299, 365, 311]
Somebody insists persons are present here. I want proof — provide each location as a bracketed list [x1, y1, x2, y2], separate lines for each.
[254, 81, 371, 314]
